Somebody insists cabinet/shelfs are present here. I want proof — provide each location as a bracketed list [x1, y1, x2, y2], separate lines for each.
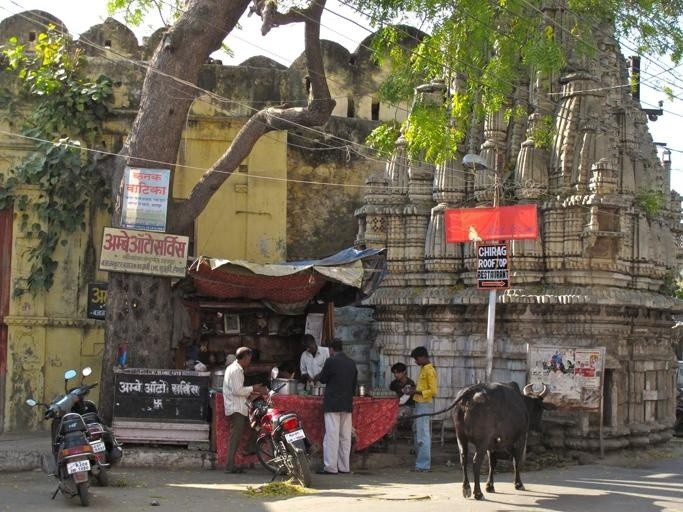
[175, 301, 334, 377]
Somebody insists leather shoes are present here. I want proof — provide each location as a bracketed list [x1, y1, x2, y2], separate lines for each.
[317, 469, 338, 475]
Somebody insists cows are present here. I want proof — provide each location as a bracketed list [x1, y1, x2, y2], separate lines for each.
[396, 380, 558, 501]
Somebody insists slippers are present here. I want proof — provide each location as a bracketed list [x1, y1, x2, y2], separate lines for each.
[409, 467, 432, 473]
[225, 467, 246, 474]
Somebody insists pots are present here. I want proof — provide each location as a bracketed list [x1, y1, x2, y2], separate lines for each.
[354, 384, 368, 396]
[310, 386, 326, 396]
[273, 378, 297, 396]
[212, 370, 224, 387]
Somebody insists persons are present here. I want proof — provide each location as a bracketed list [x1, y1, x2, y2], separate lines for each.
[300, 332, 330, 395]
[317, 337, 358, 475]
[223, 347, 260, 473]
[405, 346, 438, 472]
[390, 362, 416, 422]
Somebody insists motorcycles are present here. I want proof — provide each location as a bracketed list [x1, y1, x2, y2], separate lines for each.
[245, 366, 312, 487]
[26, 367, 123, 507]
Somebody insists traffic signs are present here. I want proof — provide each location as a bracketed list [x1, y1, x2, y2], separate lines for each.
[476, 243, 511, 290]
